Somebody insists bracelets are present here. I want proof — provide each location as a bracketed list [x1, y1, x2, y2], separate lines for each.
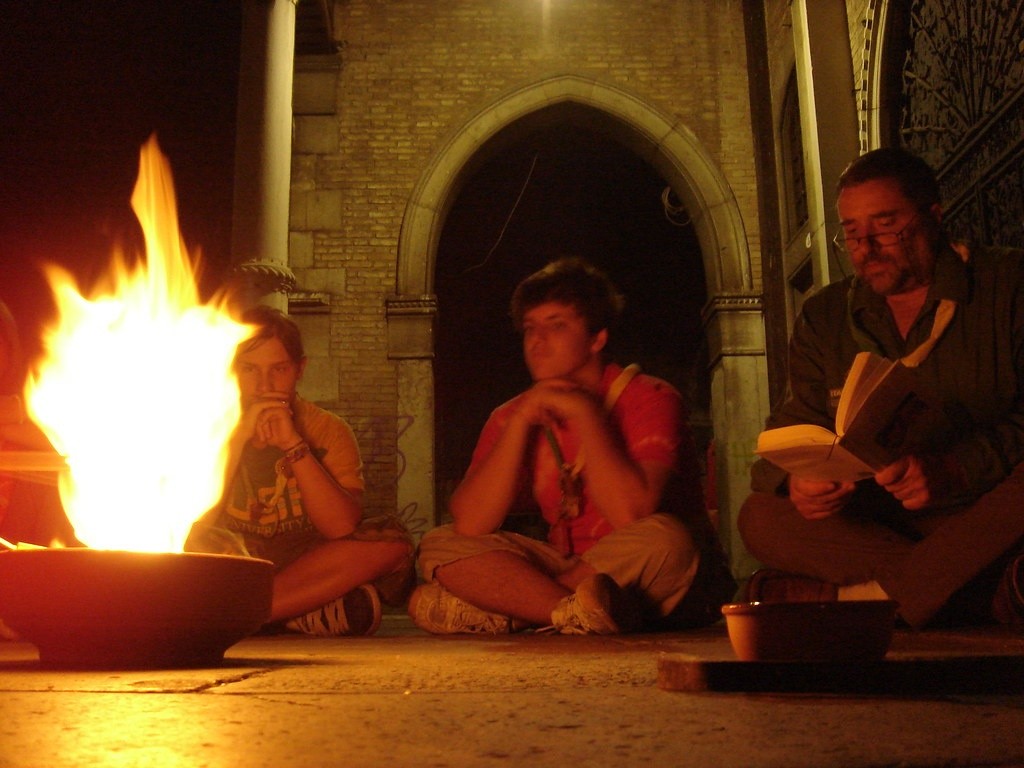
[285, 439, 309, 463]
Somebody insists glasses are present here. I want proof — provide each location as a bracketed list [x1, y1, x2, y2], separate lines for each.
[833, 209, 924, 251]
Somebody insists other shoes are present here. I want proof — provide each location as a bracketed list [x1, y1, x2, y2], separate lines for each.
[746, 569, 836, 603]
[989, 555, 1024, 625]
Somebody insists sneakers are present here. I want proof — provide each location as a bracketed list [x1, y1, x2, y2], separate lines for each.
[287, 582, 380, 637]
[535, 573, 640, 636]
[409, 584, 511, 635]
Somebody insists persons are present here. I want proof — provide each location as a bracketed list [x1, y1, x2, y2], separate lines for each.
[184, 306, 416, 635]
[408, 257, 699, 635]
[736, 147, 1024, 630]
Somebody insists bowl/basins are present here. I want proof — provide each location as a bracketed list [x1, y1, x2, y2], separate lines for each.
[721, 601, 901, 661]
[0, 549, 277, 663]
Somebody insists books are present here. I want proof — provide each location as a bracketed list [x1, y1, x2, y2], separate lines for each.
[754, 352, 918, 487]
